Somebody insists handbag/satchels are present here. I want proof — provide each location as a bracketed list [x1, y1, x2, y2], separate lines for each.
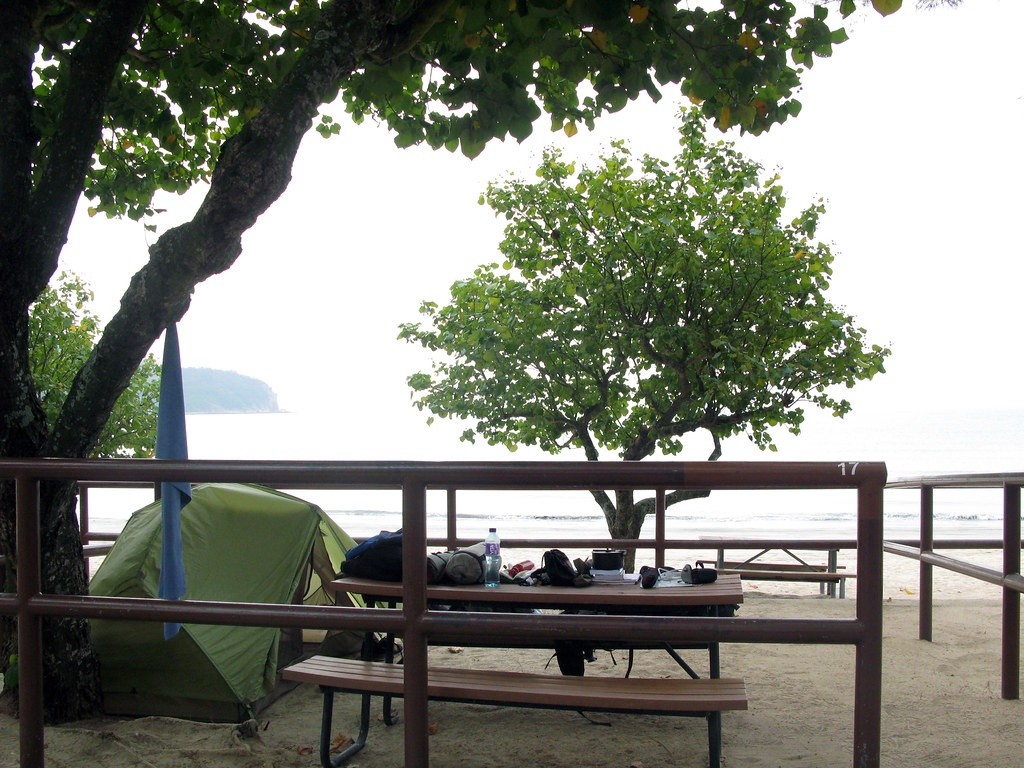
[543, 550, 573, 589]
[340, 526, 404, 583]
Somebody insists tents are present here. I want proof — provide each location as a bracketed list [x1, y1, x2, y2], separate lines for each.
[84, 481, 404, 724]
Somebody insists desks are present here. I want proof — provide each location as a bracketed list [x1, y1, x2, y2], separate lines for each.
[696, 560, 846, 573]
[329, 575, 743, 768]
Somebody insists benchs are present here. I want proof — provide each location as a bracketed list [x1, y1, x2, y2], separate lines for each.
[282, 655, 747, 768]
[699, 572, 858, 599]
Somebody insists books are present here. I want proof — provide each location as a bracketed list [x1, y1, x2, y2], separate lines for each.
[590, 568, 642, 585]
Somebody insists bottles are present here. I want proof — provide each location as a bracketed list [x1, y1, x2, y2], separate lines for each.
[509, 560, 535, 577]
[485, 528, 501, 589]
